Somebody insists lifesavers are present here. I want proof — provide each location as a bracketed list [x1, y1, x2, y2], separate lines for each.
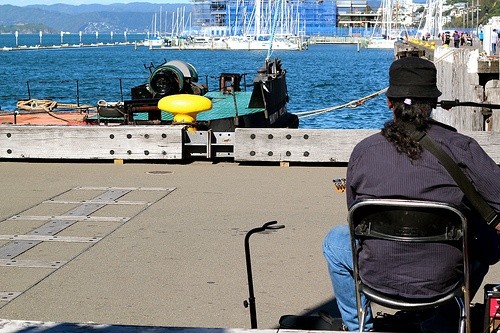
[97, 106, 126, 117]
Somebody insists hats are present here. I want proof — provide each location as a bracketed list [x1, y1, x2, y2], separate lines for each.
[385, 57, 442, 96]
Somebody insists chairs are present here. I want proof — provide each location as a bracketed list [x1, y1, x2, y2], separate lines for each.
[347, 196, 472, 333]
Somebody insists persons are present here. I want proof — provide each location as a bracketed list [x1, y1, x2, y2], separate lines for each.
[322, 56, 500, 333]
[423, 29, 500, 55]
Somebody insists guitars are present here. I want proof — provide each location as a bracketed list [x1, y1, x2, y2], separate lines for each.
[333, 177, 500, 265]
